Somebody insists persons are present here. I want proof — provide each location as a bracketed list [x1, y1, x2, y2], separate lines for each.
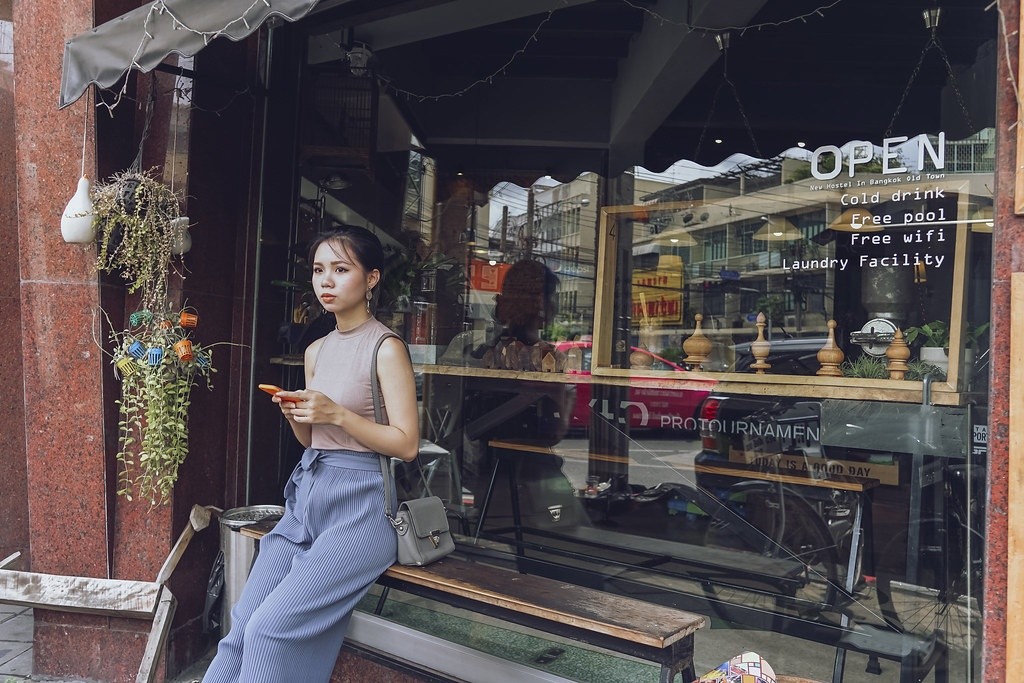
[199, 223, 420, 683]
[465, 257, 592, 530]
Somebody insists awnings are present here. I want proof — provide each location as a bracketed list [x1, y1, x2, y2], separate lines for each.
[350, 0, 1010, 199]
[61, 0, 314, 107]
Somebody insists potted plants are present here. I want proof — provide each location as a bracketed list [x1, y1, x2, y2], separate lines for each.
[902, 319, 991, 389]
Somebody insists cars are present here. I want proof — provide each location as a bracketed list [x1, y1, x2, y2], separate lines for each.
[693, 340, 858, 500]
[551, 341, 719, 433]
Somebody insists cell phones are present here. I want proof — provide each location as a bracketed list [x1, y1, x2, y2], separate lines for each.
[258, 384, 305, 404]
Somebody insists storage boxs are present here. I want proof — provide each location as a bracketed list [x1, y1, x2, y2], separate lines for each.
[409, 344, 448, 365]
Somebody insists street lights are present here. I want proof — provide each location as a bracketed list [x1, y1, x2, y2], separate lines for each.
[465, 254, 496, 303]
[761, 216, 782, 234]
[525, 199, 590, 260]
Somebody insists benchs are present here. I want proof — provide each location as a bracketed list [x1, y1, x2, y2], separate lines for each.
[375, 555, 706, 683]
[469, 443, 884, 683]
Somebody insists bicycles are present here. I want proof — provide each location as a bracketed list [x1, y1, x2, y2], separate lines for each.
[876, 373, 985, 660]
[697, 397, 873, 646]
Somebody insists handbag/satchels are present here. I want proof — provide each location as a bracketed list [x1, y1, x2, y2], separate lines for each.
[395, 496, 455, 566]
[526, 469, 581, 533]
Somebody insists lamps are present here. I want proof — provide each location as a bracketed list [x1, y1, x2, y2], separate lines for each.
[828, 207, 884, 233]
[649, 223, 699, 247]
[752, 218, 805, 241]
